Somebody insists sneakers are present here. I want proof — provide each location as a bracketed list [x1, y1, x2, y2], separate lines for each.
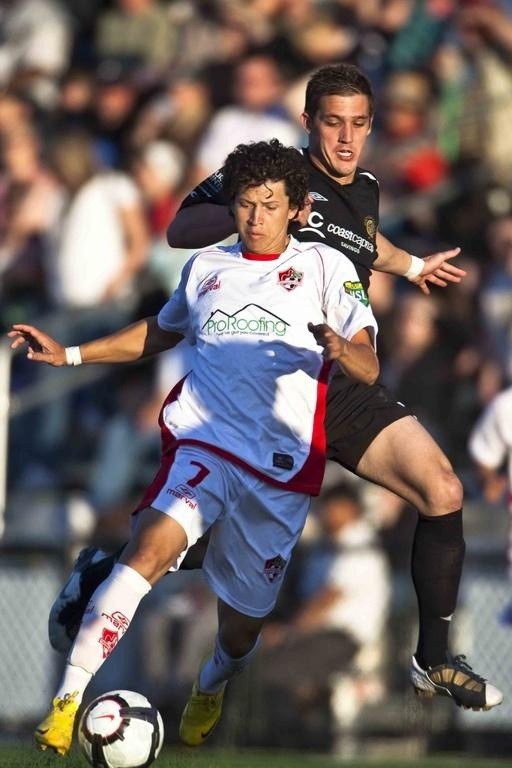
[46, 543, 114, 651]
[406, 649, 503, 711]
[32, 689, 83, 760]
[177, 649, 229, 748]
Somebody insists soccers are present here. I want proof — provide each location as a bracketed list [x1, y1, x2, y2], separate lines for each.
[78, 690, 164, 768]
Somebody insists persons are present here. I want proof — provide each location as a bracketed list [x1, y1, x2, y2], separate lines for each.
[0, 2, 512, 547]
[3, 136, 383, 758]
[215, 478, 398, 751]
[42, 59, 506, 713]
